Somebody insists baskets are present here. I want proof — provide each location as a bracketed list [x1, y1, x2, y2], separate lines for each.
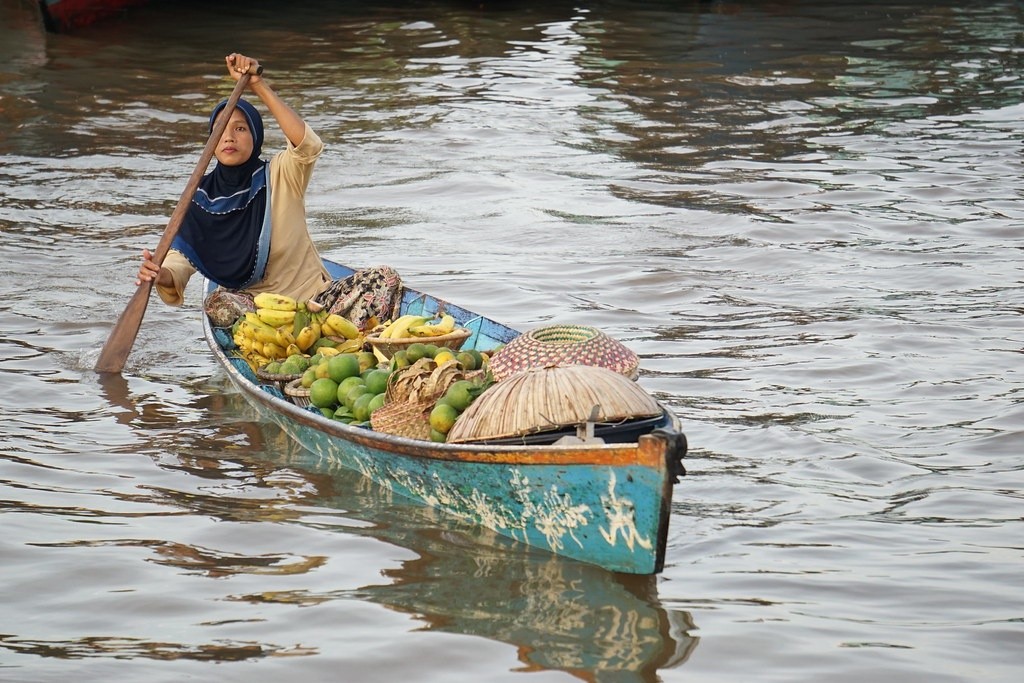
[284, 378, 311, 408]
[257, 360, 302, 395]
[367, 327, 473, 362]
[369, 358, 483, 443]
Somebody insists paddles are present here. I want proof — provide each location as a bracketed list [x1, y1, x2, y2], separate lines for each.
[93, 62, 264, 374]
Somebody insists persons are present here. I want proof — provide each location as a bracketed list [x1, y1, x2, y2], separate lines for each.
[134, 53, 403, 335]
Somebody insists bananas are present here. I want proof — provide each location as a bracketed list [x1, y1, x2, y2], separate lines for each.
[370, 309, 454, 340]
[231, 292, 359, 367]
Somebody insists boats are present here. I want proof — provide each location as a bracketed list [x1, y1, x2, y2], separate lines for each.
[201, 256, 687, 574]
